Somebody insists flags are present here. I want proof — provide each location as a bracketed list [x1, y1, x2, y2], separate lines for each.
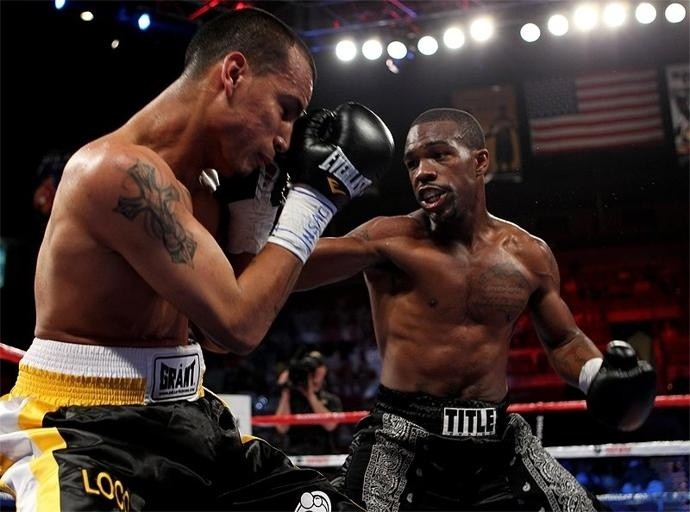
[519, 68, 663, 152]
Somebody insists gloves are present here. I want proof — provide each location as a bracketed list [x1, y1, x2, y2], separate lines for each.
[218, 161, 291, 209]
[582, 338, 659, 434]
[289, 98, 394, 214]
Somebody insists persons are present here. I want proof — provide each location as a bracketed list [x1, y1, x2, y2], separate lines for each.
[222, 330, 381, 453]
[0, 10, 394, 512]
[577, 454, 690, 512]
[193, 106, 655, 512]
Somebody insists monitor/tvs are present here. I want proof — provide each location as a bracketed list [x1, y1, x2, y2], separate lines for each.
[287, 344, 318, 388]
[658, 59, 690, 167]
[448, 85, 529, 185]
